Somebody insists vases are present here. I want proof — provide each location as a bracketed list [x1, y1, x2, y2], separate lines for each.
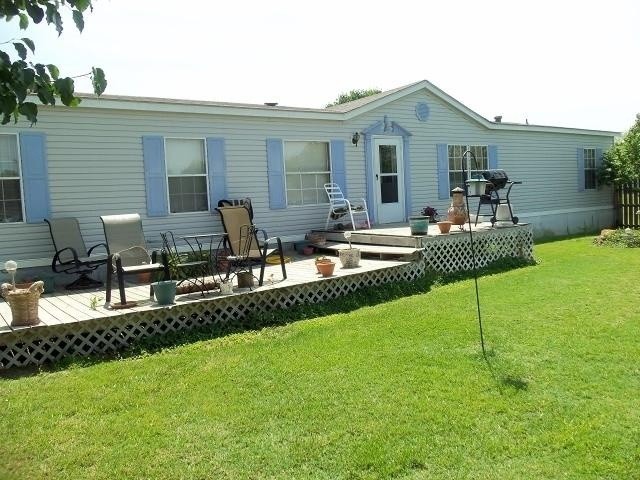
[409, 216, 430, 235]
[436, 221, 452, 233]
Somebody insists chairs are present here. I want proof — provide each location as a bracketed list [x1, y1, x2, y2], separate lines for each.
[324, 183, 371, 231]
[44, 212, 208, 308]
[213, 206, 287, 287]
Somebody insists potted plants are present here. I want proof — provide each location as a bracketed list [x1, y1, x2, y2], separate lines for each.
[315, 257, 335, 276]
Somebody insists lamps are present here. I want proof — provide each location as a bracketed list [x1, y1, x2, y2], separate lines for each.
[462, 148, 490, 360]
[352, 132, 361, 146]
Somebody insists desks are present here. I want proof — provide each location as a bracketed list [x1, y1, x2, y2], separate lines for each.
[179, 230, 229, 297]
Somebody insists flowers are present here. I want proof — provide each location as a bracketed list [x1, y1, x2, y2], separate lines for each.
[421, 208, 438, 222]
